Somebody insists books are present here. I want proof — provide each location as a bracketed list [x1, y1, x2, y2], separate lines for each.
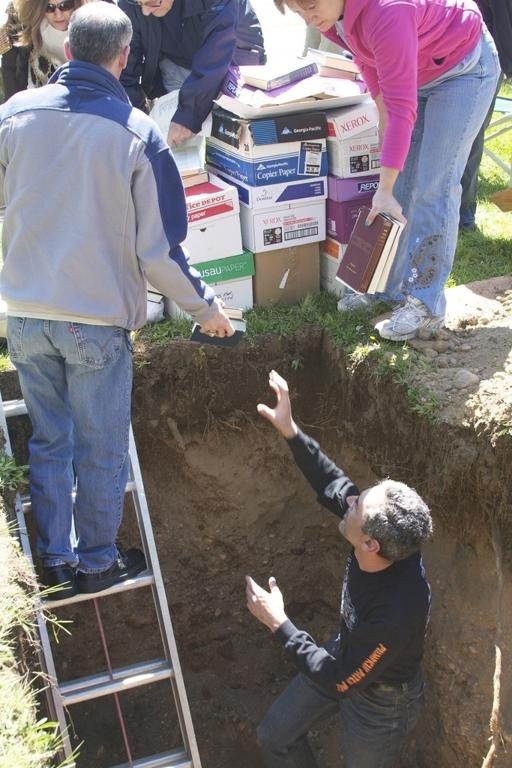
[190, 304, 247, 348]
[170, 171, 210, 191]
[238, 44, 367, 95]
[336, 205, 406, 294]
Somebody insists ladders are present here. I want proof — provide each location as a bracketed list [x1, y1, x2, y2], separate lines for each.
[0, 398, 202, 768]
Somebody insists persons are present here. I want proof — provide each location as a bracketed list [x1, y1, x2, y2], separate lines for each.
[274, 0, 503, 342]
[458, 1, 512, 231]
[1, 0, 235, 603]
[115, 1, 267, 149]
[0, 0, 86, 103]
[243, 368, 433, 768]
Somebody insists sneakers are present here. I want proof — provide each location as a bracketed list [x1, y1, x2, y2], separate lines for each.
[77, 546, 147, 595]
[42, 566, 77, 601]
[336, 292, 378, 312]
[375, 295, 446, 341]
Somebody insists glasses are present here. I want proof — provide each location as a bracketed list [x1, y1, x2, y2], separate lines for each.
[44, 0, 76, 13]
[125, 1, 163, 7]
